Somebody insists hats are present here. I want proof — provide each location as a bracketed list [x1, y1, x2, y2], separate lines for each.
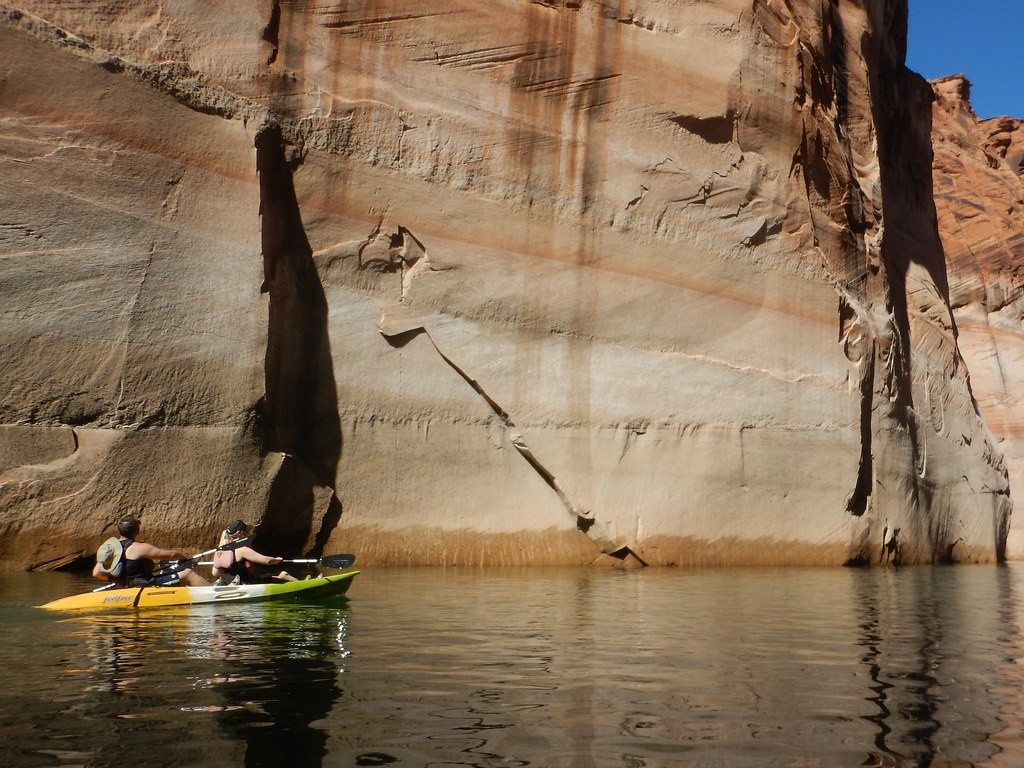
[96, 536, 124, 575]
[226, 520, 253, 537]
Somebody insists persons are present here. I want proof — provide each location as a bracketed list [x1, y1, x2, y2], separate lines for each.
[212, 522, 323, 586]
[92, 515, 240, 589]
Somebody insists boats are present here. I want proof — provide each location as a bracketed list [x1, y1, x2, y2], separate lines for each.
[33, 569, 362, 615]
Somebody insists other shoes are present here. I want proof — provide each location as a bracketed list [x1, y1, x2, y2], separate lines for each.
[315, 573, 323, 578]
[305, 575, 311, 580]
[228, 574, 241, 586]
[212, 576, 222, 586]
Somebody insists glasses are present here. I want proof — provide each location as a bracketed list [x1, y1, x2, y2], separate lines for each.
[137, 520, 141, 525]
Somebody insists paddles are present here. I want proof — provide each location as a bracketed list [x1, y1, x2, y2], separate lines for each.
[158, 552, 357, 574]
[89, 531, 258, 593]
